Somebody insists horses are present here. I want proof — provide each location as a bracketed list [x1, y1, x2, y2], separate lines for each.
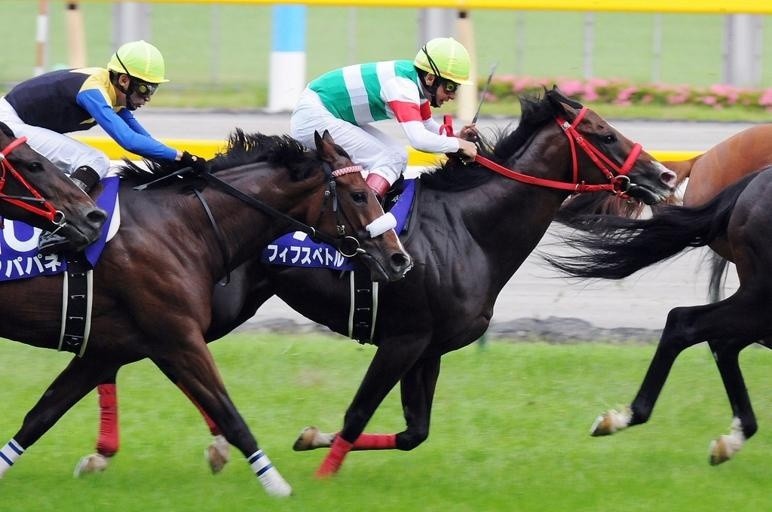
[560, 121, 772, 210]
[0, 116, 110, 250]
[0, 124, 416, 501]
[539, 164, 771, 468]
[75, 82, 679, 486]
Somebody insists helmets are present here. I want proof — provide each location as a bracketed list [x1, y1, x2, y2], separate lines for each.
[107, 38, 169, 85]
[414, 37, 473, 86]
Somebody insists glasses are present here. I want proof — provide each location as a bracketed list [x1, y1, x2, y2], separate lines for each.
[133, 80, 159, 96]
[442, 79, 460, 94]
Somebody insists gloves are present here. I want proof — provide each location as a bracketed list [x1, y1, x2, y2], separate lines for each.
[180, 150, 207, 171]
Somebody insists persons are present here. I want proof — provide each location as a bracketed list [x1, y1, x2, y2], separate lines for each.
[0, 40, 205, 198]
[291, 36, 479, 203]
